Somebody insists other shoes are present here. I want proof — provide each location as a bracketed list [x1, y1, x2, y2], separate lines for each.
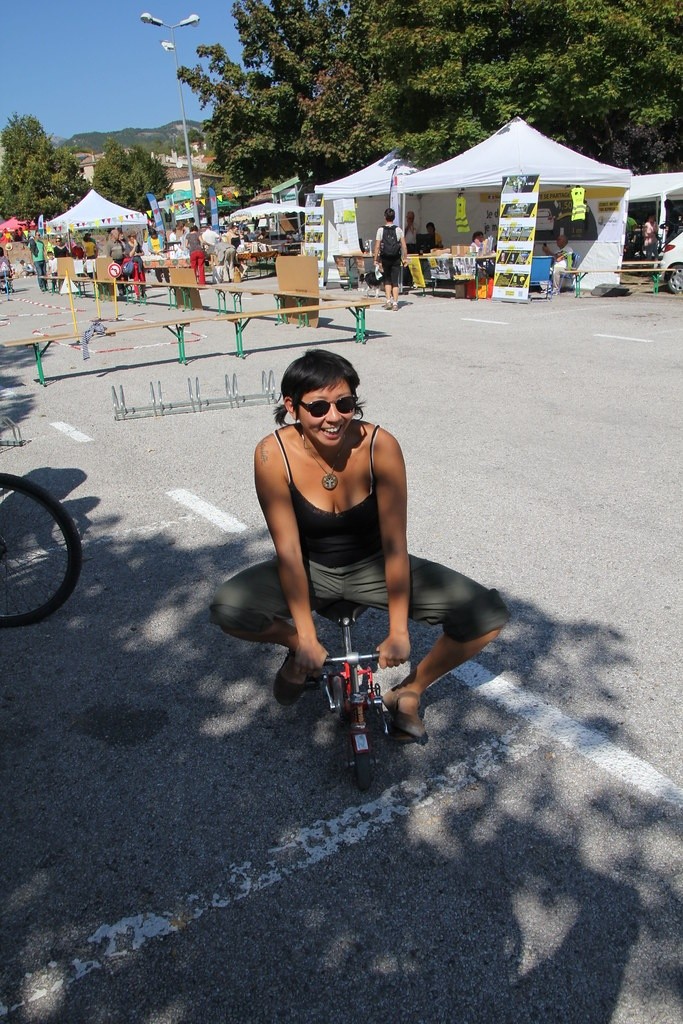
[382, 301, 392, 310]
[389, 302, 399, 311]
[382, 684, 426, 739]
[272, 647, 308, 706]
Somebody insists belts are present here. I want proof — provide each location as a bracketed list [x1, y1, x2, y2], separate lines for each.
[87, 256, 95, 259]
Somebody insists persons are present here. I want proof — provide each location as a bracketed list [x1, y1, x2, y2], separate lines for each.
[542, 235, 573, 296]
[0, 222, 271, 299]
[642, 217, 659, 261]
[404, 211, 416, 254]
[426, 221, 442, 249]
[471, 231, 494, 279]
[373, 208, 408, 311]
[208, 350, 511, 738]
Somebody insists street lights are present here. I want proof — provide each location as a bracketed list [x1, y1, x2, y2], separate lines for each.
[140, 12, 201, 229]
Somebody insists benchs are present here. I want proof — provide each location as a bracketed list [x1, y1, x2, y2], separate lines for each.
[0, 276, 384, 385]
[565, 269, 677, 298]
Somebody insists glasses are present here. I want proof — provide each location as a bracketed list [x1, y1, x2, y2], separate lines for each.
[35, 234, 41, 236]
[47, 251, 52, 254]
[55, 240, 61, 243]
[288, 390, 359, 418]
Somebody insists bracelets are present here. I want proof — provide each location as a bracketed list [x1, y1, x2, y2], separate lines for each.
[374, 260, 378, 261]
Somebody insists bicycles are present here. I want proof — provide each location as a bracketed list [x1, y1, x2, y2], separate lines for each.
[303, 597, 380, 791]
[0, 471, 83, 626]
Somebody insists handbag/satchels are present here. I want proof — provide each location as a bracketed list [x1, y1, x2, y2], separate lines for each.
[118, 260, 135, 277]
[211, 264, 230, 284]
[233, 266, 244, 283]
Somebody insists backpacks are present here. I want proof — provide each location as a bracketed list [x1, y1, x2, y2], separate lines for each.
[379, 225, 402, 261]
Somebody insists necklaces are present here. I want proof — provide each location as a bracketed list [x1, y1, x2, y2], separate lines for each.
[302, 436, 346, 490]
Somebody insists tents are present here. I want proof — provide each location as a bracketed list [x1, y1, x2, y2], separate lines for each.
[0, 216, 38, 231]
[313, 147, 448, 290]
[45, 188, 148, 257]
[230, 201, 306, 244]
[629, 172, 682, 262]
[390, 116, 632, 293]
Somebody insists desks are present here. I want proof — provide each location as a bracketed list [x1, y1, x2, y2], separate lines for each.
[237, 250, 293, 279]
[405, 254, 496, 300]
[143, 258, 191, 269]
[333, 253, 376, 288]
[622, 260, 664, 268]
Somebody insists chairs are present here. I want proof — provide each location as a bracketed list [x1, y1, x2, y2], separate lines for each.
[558, 252, 581, 292]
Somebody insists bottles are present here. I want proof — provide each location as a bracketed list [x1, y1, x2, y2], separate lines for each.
[364, 242, 370, 255]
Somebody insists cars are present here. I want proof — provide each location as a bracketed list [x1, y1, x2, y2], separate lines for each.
[654, 221, 683, 294]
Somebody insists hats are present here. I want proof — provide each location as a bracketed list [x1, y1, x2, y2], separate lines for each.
[125, 230, 139, 237]
[85, 233, 91, 237]
[5, 242, 13, 251]
